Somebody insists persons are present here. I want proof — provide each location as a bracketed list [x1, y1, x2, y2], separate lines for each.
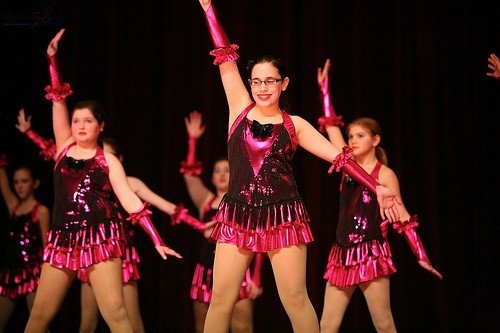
[179, 110, 262, 333]
[317, 58, 442, 333]
[487, 53, 500, 78]
[200, 0, 402, 333]
[0, 28, 219, 333]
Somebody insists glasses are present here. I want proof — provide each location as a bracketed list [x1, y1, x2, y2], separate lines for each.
[248, 79, 284, 86]
